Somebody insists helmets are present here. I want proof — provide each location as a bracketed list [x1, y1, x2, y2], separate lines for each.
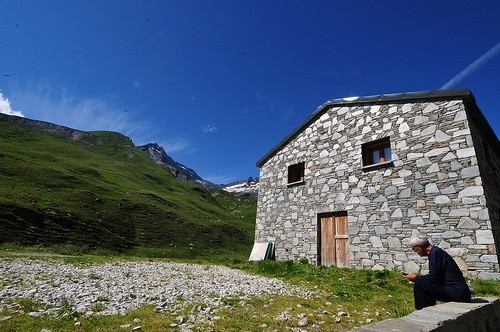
[410, 234, 430, 250]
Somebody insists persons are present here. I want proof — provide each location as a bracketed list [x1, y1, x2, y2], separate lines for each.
[406, 236, 471, 310]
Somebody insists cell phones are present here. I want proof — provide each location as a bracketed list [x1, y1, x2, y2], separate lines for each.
[402, 272, 408, 276]
